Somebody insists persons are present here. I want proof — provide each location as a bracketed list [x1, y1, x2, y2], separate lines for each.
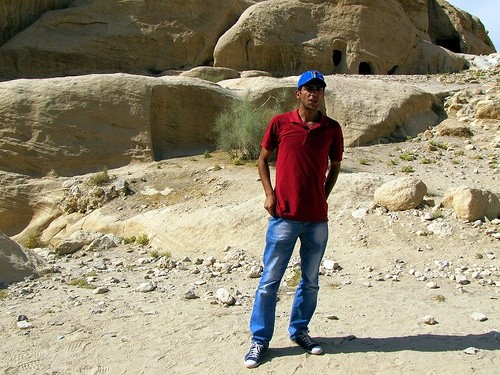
[243, 70, 345, 367]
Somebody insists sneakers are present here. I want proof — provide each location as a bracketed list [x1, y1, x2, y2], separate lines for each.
[289, 331, 324, 355]
[243, 342, 269, 369]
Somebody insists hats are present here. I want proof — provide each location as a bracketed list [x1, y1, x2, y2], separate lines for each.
[297, 69, 326, 90]
